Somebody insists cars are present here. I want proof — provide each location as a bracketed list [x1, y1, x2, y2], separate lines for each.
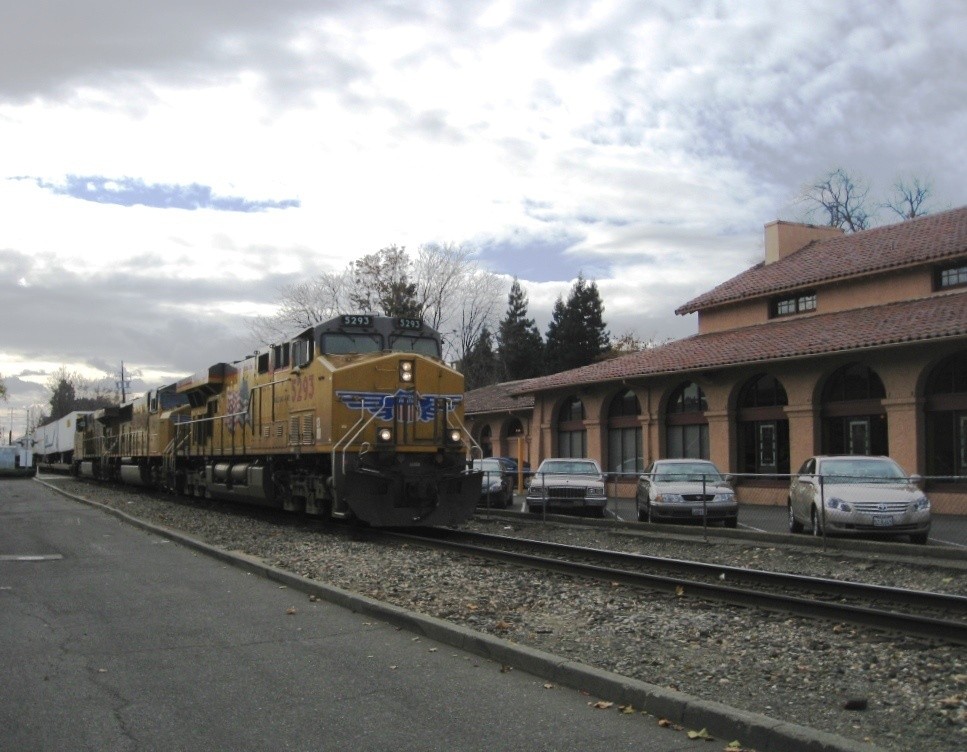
[526, 458, 608, 518]
[787, 456, 932, 545]
[635, 459, 738, 528]
[616, 457, 643, 479]
[465, 458, 514, 508]
[487, 456, 530, 489]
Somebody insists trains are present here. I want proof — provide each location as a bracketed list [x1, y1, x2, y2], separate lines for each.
[30, 314, 484, 528]
